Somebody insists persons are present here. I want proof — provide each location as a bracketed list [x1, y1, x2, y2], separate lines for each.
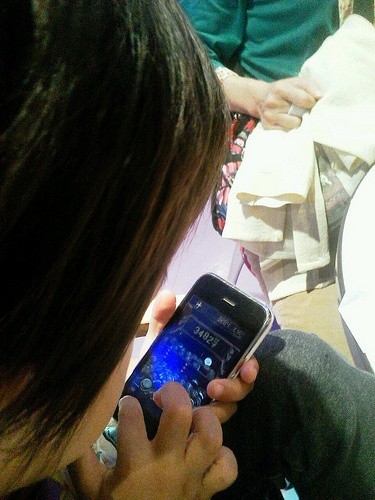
[1, 0, 374, 500]
[176, 1, 375, 247]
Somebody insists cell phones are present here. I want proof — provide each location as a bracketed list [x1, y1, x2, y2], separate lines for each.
[113, 270, 273, 442]
